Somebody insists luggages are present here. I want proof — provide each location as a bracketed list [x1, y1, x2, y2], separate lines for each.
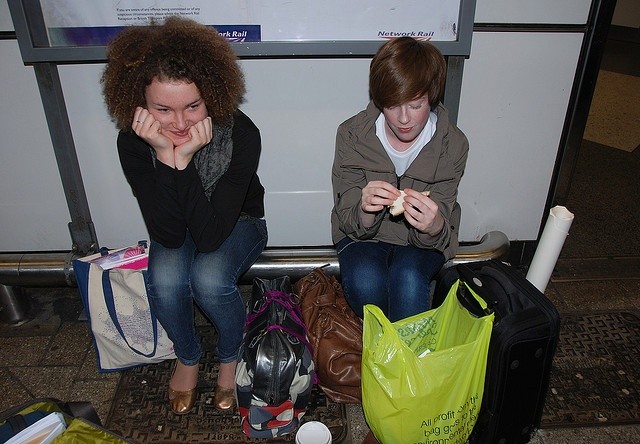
[432, 257, 562, 444]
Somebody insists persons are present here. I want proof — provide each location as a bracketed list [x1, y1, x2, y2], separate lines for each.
[331, 37, 469, 323]
[98, 14, 268, 416]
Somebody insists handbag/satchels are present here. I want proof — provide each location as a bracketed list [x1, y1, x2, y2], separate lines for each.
[73, 238, 178, 373]
[233, 274, 321, 440]
[1, 398, 133, 444]
[293, 263, 363, 405]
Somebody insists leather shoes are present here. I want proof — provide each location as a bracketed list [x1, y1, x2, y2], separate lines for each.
[215, 367, 236, 412]
[168, 358, 198, 413]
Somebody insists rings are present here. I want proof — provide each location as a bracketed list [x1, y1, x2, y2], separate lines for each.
[137, 120, 144, 124]
